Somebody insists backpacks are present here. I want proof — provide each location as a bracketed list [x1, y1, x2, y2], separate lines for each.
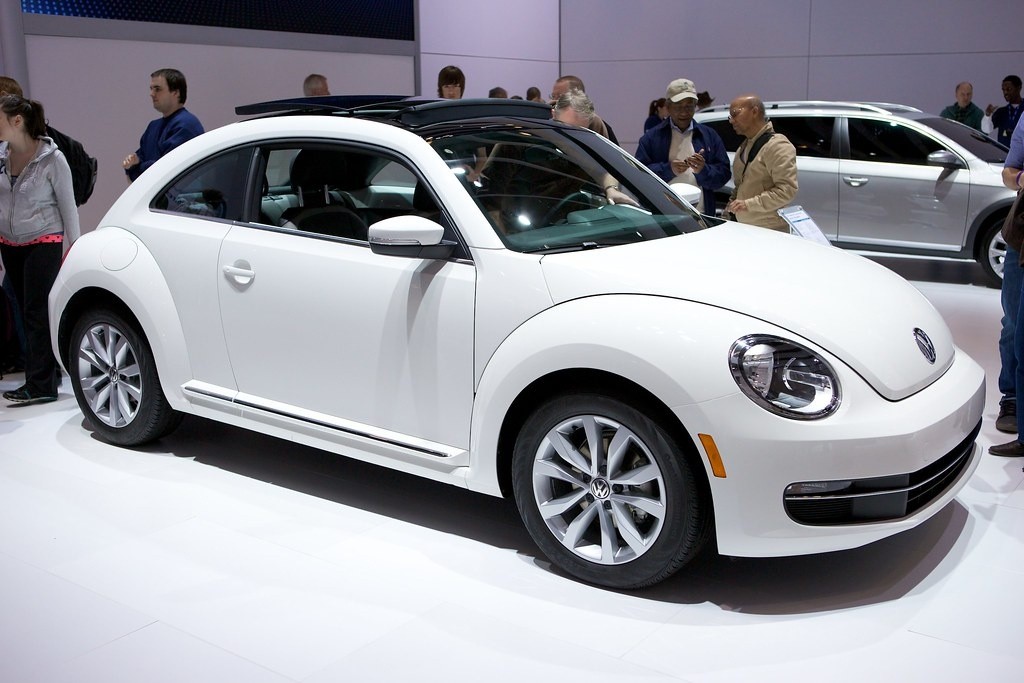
[47, 125, 99, 209]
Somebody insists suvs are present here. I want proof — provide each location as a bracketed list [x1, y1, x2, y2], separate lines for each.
[691, 99, 1017, 288]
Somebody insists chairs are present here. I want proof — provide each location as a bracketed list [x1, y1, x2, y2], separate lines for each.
[279, 148, 368, 245]
[413, 162, 476, 224]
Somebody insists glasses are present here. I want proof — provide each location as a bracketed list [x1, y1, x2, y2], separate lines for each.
[727, 108, 745, 124]
[548, 92, 565, 100]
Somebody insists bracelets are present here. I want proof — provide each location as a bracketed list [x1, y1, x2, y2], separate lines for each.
[604, 184, 621, 192]
[1016, 171, 1024, 187]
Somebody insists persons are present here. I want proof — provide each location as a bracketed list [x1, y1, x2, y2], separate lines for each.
[634, 78, 732, 229]
[988, 239, 1024, 474]
[937, 82, 988, 136]
[476, 87, 640, 234]
[694, 91, 715, 113]
[981, 75, 1024, 148]
[643, 97, 669, 133]
[716, 94, 799, 233]
[488, 87, 508, 99]
[122, 68, 204, 183]
[996, 109, 1024, 434]
[549, 75, 620, 147]
[303, 73, 330, 95]
[0, 77, 80, 404]
[426, 64, 487, 180]
[526, 88, 540, 102]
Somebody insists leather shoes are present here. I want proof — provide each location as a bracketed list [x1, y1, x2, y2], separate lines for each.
[989, 440, 1024, 458]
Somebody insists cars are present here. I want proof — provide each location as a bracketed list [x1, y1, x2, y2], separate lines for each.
[46, 88, 987, 591]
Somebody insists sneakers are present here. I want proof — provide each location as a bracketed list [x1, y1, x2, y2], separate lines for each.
[56, 369, 63, 386]
[996, 402, 1018, 432]
[3, 382, 60, 404]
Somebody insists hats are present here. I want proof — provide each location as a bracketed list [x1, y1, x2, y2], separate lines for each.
[696, 91, 716, 106]
[665, 78, 699, 103]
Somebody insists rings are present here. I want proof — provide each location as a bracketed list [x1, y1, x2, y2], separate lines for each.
[695, 164, 698, 168]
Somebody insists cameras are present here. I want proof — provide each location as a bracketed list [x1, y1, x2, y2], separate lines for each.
[715, 206, 736, 221]
